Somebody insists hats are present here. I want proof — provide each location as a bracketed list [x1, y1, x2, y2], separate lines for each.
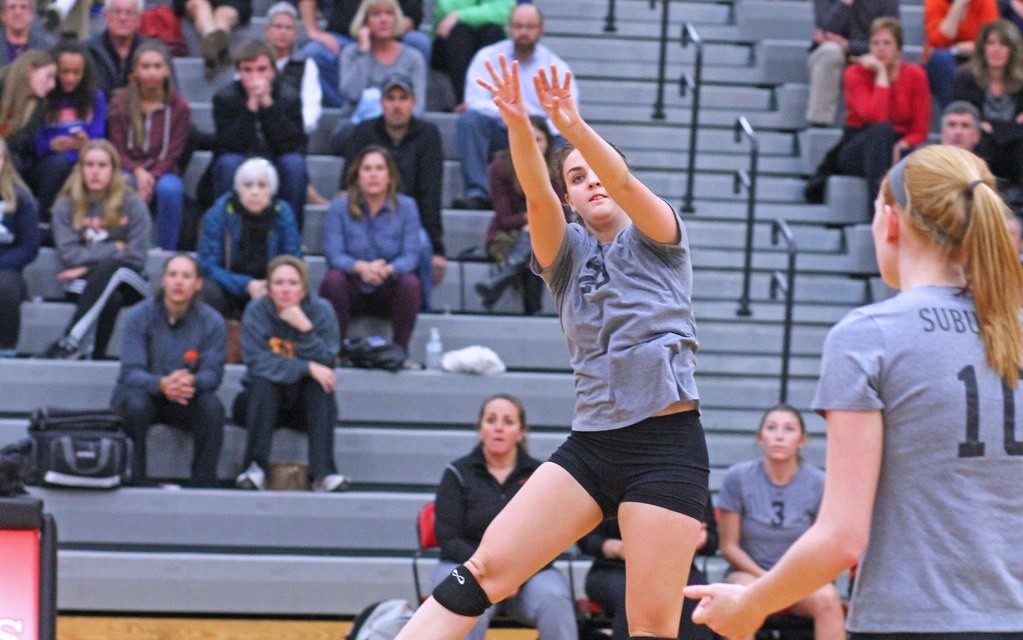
[381, 71, 413, 96]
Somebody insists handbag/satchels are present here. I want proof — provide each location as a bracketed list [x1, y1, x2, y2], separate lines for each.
[24, 407, 134, 491]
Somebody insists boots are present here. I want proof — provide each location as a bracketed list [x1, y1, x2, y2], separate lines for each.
[475, 230, 542, 313]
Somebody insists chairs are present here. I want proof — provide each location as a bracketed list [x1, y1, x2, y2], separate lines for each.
[410, 502, 579, 617]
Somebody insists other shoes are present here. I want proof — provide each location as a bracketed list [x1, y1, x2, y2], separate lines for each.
[451, 195, 490, 210]
[36, 340, 84, 360]
[238, 463, 267, 490]
[201, 27, 234, 80]
[311, 475, 348, 494]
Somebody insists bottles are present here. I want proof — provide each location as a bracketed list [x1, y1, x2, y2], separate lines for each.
[424, 328, 442, 371]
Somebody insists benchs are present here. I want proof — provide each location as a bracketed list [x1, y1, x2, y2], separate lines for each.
[730, 0, 1023, 307]
[0, 0, 595, 620]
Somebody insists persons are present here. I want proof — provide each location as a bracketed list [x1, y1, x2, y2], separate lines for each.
[48, 139, 150, 360]
[0, 0, 1023, 640]
[315, 144, 420, 369]
[838, 16, 930, 221]
[195, 156, 303, 323]
[232, 254, 353, 492]
[107, 42, 193, 252]
[105, 254, 227, 487]
[393, 54, 710, 640]
